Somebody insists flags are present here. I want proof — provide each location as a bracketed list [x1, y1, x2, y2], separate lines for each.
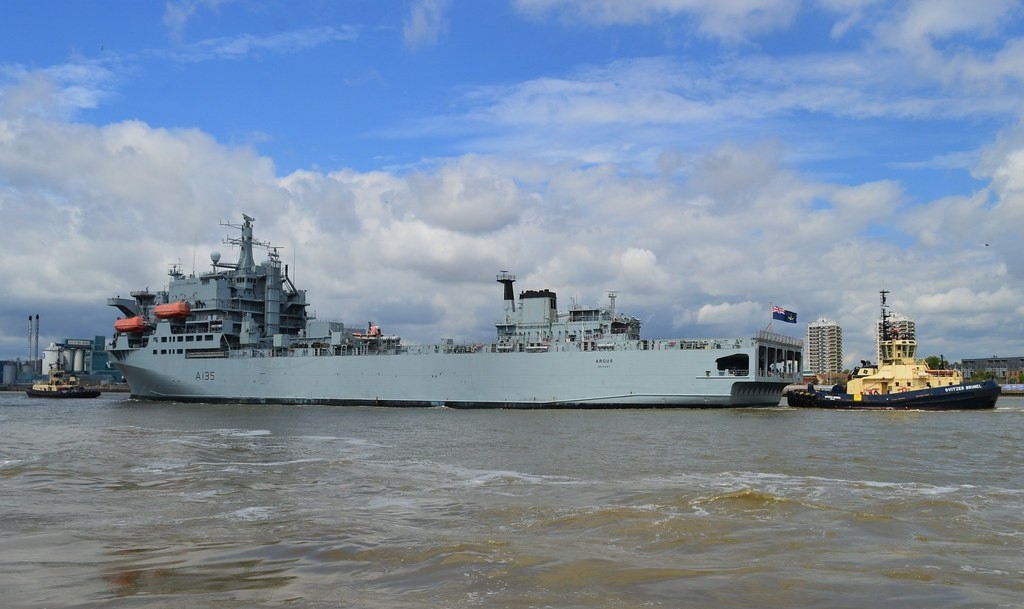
[770, 305, 797, 323]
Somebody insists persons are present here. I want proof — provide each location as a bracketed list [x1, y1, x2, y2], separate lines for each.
[890, 326, 899, 340]
[636, 338, 739, 351]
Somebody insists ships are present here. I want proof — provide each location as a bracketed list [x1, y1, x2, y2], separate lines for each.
[106, 214, 790, 410]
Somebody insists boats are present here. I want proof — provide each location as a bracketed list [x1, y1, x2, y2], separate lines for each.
[114, 315, 144, 332]
[25, 348, 102, 398]
[787, 289, 1002, 408]
[154, 302, 191, 319]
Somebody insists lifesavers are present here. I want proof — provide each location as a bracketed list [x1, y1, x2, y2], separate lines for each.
[471, 348, 475, 353]
[789, 392, 812, 402]
[768, 371, 772, 377]
[912, 368, 916, 374]
[902, 387, 907, 392]
